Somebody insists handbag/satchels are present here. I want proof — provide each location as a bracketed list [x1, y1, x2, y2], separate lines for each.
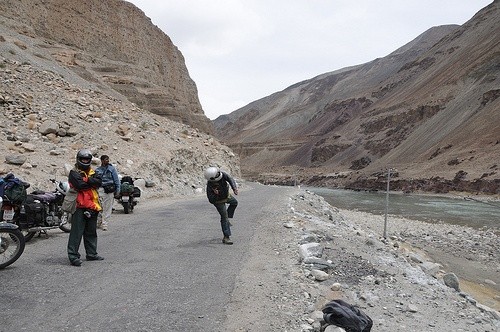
[24, 203, 44, 223]
[61, 188, 77, 214]
[121, 182, 134, 192]
[103, 183, 117, 194]
[0, 174, 27, 204]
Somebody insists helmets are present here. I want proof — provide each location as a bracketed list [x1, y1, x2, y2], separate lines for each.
[77, 149, 94, 169]
[204, 167, 223, 181]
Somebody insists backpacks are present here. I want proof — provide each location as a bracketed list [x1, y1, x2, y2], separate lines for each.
[321, 300, 373, 332]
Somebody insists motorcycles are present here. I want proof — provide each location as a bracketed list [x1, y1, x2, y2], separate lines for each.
[113, 175, 141, 214]
[0, 172, 73, 270]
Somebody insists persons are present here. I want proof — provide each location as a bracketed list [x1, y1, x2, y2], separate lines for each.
[94, 155, 120, 231]
[204, 167, 238, 245]
[67, 150, 104, 266]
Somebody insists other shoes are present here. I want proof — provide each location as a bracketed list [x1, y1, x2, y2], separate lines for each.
[86, 253, 104, 261]
[219, 219, 232, 226]
[72, 259, 83, 266]
[222, 236, 233, 245]
[103, 226, 107, 231]
[97, 220, 102, 228]
